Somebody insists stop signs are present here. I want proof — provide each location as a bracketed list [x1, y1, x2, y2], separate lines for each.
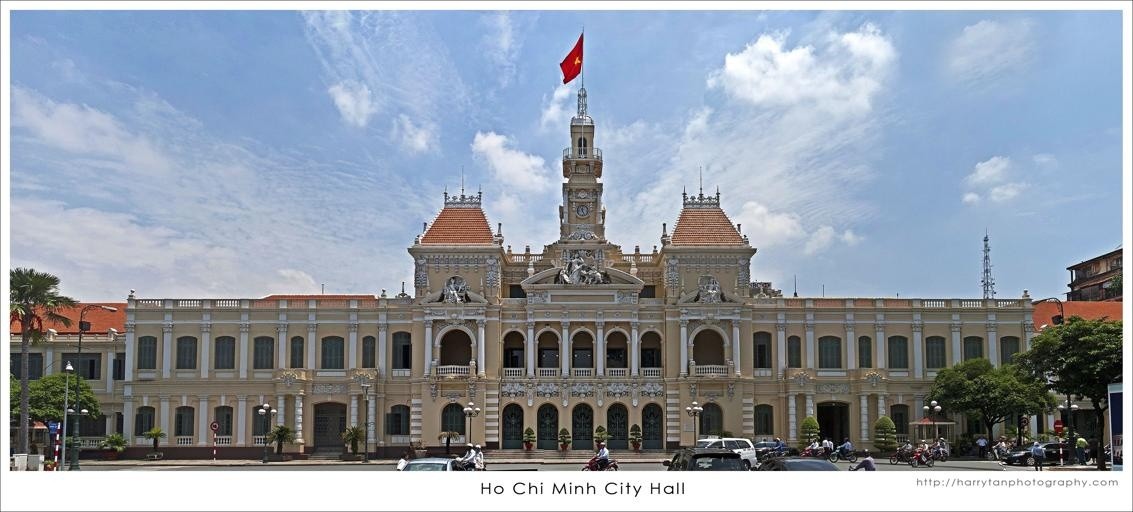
[1053, 419, 1063, 433]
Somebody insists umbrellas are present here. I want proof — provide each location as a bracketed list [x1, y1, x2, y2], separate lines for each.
[908, 413, 959, 439]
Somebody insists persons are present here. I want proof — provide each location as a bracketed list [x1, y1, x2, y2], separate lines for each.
[822, 437, 830, 460]
[472, 444, 485, 471]
[593, 442, 610, 470]
[771, 437, 784, 457]
[1031, 441, 1046, 471]
[900, 439, 913, 462]
[458, 442, 475, 471]
[568, 253, 584, 272]
[929, 438, 940, 460]
[838, 437, 852, 460]
[991, 435, 1029, 461]
[808, 438, 819, 457]
[580, 266, 597, 285]
[447, 276, 462, 303]
[917, 439, 930, 460]
[701, 278, 720, 302]
[1061, 431, 1098, 465]
[976, 435, 988, 458]
[395, 451, 410, 471]
[848, 448, 876, 471]
[828, 437, 834, 456]
[938, 437, 945, 456]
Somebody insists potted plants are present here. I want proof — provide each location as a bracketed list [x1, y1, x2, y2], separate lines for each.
[99, 432, 128, 460]
[438, 431, 459, 458]
[557, 429, 572, 450]
[340, 426, 365, 461]
[413, 440, 427, 458]
[521, 427, 536, 450]
[143, 427, 167, 460]
[268, 423, 296, 461]
[628, 424, 643, 450]
[42, 460, 53, 471]
[594, 426, 608, 449]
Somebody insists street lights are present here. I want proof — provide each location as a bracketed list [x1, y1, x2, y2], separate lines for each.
[58, 359, 72, 470]
[70, 304, 118, 470]
[359, 382, 370, 462]
[1059, 399, 1079, 412]
[685, 400, 703, 448]
[922, 400, 941, 444]
[1031, 297, 1077, 464]
[258, 404, 277, 463]
[462, 401, 480, 446]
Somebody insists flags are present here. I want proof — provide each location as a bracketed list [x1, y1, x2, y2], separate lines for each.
[559, 32, 584, 85]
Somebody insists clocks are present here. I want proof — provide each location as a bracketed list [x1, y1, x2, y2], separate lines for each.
[577, 205, 588, 216]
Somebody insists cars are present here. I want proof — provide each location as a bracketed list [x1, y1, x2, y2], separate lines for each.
[662, 437, 841, 471]
[398, 457, 466, 471]
[1002, 441, 1070, 466]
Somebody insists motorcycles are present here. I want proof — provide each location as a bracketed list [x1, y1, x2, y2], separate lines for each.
[801, 447, 857, 463]
[581, 454, 619, 470]
[456, 458, 486, 471]
[890, 447, 949, 468]
